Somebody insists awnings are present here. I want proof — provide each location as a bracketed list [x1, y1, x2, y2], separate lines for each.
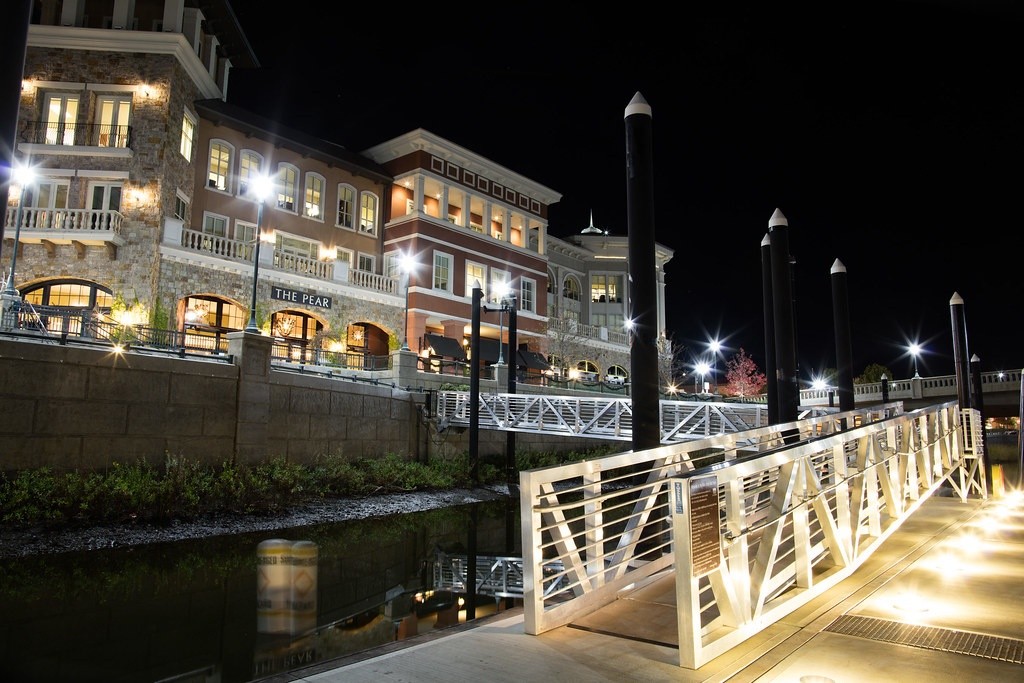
[479, 337, 550, 370]
[425, 333, 467, 358]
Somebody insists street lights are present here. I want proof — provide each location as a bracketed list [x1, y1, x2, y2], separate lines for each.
[4, 169, 31, 295]
[910, 346, 919, 377]
[244, 177, 269, 334]
[712, 342, 719, 395]
[495, 283, 508, 364]
[400, 256, 414, 352]
[699, 365, 706, 393]
